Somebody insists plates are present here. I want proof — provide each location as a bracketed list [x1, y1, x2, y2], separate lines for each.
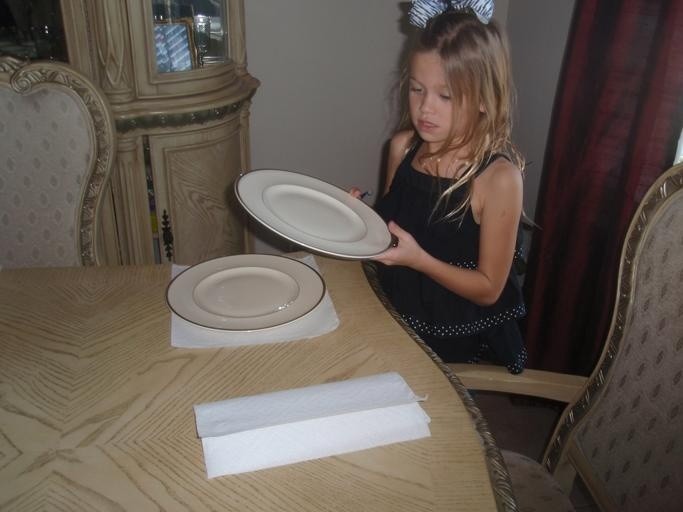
[165, 253, 327, 334]
[233, 167, 395, 261]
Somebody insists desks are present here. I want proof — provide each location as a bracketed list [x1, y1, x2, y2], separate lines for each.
[0, 249, 517, 512]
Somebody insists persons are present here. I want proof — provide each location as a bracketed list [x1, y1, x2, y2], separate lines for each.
[347, 0, 531, 398]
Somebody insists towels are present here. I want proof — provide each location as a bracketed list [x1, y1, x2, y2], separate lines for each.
[190, 370, 430, 481]
[166, 254, 341, 349]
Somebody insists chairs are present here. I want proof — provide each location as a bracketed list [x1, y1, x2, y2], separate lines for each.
[446, 160, 683, 512]
[1, 53, 120, 272]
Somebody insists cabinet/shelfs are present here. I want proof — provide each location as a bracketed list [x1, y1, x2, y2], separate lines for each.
[0, 0, 262, 267]
